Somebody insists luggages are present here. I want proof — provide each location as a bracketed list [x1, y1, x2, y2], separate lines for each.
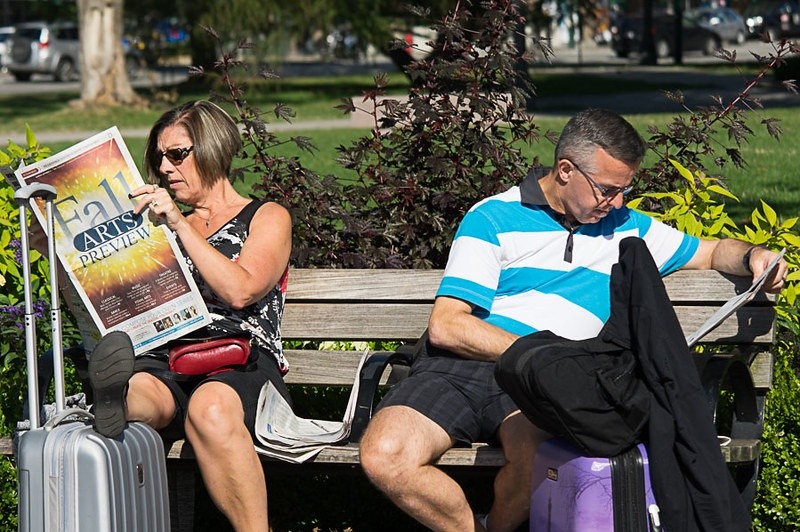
[13, 181, 171, 531]
[526, 433, 664, 532]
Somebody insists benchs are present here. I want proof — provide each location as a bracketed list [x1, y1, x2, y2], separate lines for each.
[159, 267, 779, 532]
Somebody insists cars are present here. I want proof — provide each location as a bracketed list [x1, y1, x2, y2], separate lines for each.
[610, 14, 723, 57]
[683, 9, 748, 45]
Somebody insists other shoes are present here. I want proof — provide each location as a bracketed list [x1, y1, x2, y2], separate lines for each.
[87, 331, 137, 439]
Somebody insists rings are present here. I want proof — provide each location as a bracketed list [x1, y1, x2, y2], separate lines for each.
[151, 200, 159, 207]
[782, 277, 786, 284]
[153, 184, 158, 192]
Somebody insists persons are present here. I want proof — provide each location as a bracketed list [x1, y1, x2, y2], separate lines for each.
[359, 107, 789, 532]
[30, 99, 293, 532]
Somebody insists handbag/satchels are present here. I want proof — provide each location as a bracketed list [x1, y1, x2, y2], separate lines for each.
[166, 329, 256, 375]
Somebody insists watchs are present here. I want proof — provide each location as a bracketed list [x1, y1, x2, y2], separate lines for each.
[742, 246, 764, 274]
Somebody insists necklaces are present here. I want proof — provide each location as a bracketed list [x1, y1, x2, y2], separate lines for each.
[194, 194, 240, 228]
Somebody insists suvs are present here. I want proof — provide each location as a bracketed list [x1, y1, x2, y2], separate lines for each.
[0, 19, 144, 85]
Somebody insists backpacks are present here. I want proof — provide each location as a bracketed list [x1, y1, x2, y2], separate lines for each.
[492, 329, 652, 456]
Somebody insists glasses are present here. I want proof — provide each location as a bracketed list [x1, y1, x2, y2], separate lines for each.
[154, 145, 196, 170]
[560, 156, 635, 201]
